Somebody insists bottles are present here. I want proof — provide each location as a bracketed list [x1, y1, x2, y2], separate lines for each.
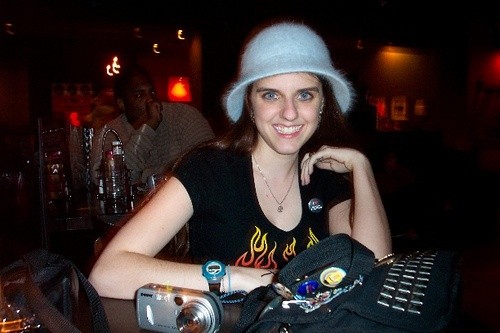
[96, 176, 107, 215]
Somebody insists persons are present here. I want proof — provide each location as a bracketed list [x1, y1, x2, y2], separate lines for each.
[87, 21, 393, 301]
[374, 132, 500, 243]
[90, 65, 218, 256]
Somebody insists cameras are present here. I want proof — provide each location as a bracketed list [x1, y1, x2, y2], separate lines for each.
[136, 283, 224, 333]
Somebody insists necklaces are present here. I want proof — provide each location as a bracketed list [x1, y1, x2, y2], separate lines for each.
[251, 150, 298, 212]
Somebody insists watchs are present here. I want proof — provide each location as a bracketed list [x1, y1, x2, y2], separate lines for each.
[202, 260, 227, 296]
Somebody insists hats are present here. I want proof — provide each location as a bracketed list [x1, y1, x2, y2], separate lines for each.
[222, 22, 351, 123]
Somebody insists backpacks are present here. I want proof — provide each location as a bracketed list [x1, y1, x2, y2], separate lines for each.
[231, 233, 464, 333]
[0, 249, 112, 333]
